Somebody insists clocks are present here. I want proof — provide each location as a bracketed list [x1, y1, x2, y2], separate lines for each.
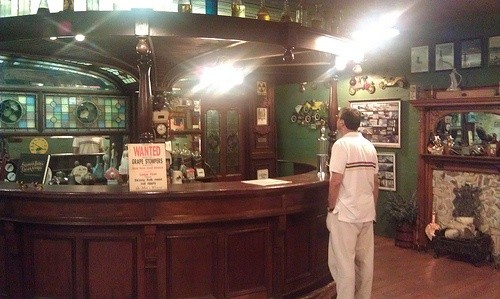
[156, 123, 168, 136]
[28, 137, 49, 154]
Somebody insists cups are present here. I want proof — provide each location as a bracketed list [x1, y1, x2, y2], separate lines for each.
[172, 170, 182, 184]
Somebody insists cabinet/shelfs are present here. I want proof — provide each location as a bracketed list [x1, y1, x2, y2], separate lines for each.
[407, 81, 500, 252]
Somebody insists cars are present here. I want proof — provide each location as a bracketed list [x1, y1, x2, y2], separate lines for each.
[379, 74, 406, 89]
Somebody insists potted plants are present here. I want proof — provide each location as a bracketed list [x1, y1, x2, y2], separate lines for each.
[380, 188, 420, 248]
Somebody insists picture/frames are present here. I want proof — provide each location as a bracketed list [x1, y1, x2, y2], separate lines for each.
[486, 34, 500, 68]
[434, 40, 456, 73]
[376, 150, 398, 193]
[409, 43, 431, 76]
[460, 36, 484, 70]
[347, 98, 402, 149]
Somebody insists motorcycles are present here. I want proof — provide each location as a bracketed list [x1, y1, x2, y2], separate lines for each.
[348, 77, 375, 95]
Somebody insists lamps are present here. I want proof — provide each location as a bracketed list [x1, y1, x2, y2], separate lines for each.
[297, 82, 307, 93]
[282, 46, 295, 64]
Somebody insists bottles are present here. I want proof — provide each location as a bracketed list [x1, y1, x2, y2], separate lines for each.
[178, 0, 191, 14]
[172, 143, 181, 170]
[181, 143, 191, 168]
[192, 144, 203, 167]
[280, 0, 346, 35]
[86, 0, 99, 11]
[256, 0, 271, 21]
[231, 0, 246, 18]
[93, 156, 105, 179]
[36, 0, 50, 14]
[316, 127, 330, 173]
[0, 0, 11, 16]
[17, 0, 31, 16]
[105, 158, 119, 180]
[63, 0, 75, 11]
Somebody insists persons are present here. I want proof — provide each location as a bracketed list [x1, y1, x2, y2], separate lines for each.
[87, 162, 93, 173]
[326, 107, 379, 299]
[69, 161, 87, 184]
[72, 136, 105, 154]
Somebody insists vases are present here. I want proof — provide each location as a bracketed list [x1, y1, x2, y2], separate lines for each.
[316, 125, 329, 175]
